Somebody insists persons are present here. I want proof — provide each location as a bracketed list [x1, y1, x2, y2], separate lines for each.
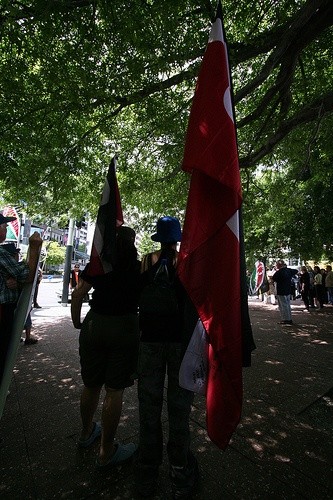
[70, 227, 142, 467]
[0, 243, 333, 344]
[139, 216, 198, 473]
[0, 214, 42, 379]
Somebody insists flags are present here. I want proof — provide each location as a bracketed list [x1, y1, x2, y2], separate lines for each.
[179, 0, 254, 450]
[87, 157, 125, 277]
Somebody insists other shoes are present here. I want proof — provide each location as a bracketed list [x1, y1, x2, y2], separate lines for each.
[25, 338, 38, 343]
[171, 456, 198, 488]
[281, 320, 292, 324]
[34, 304, 41, 309]
[78, 422, 101, 445]
[96, 442, 137, 471]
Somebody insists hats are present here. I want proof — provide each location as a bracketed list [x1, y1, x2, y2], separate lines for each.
[151, 216, 188, 242]
[0, 213, 16, 224]
